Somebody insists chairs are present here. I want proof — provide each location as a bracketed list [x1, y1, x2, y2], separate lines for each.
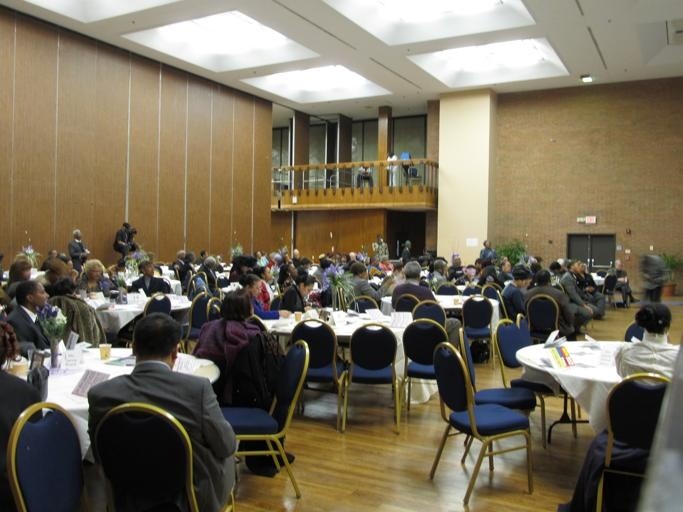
[2, 255, 629, 404]
[93, 403, 236, 512]
[494, 317, 578, 449]
[624, 321, 671, 343]
[430, 342, 535, 506]
[290, 318, 347, 431]
[400, 318, 449, 427]
[6, 400, 87, 511]
[342, 322, 402, 434]
[219, 339, 310, 499]
[458, 327, 536, 471]
[596, 371, 671, 512]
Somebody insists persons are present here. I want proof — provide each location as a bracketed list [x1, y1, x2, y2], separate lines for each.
[113, 222, 141, 259]
[639, 253, 668, 303]
[4, 249, 636, 345]
[402, 241, 411, 265]
[372, 234, 389, 261]
[190, 287, 286, 415]
[592, 302, 681, 473]
[68, 230, 90, 274]
[480, 240, 498, 267]
[384, 150, 400, 187]
[0, 320, 52, 511]
[355, 162, 374, 188]
[4, 279, 59, 354]
[84, 312, 238, 511]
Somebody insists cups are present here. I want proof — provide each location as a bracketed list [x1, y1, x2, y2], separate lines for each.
[227, 281, 240, 291]
[389, 311, 405, 329]
[292, 310, 302, 324]
[161, 265, 168, 275]
[8, 359, 28, 376]
[331, 310, 347, 327]
[26, 346, 42, 364]
[96, 342, 111, 362]
[302, 305, 314, 315]
[452, 296, 459, 306]
[62, 348, 83, 374]
[78, 283, 183, 311]
[30, 267, 37, 279]
[194, 360, 215, 377]
[312, 282, 318, 291]
[464, 280, 470, 288]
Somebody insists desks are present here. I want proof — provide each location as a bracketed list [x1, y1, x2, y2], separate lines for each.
[1, 345, 220, 462]
[515, 339, 683, 437]
